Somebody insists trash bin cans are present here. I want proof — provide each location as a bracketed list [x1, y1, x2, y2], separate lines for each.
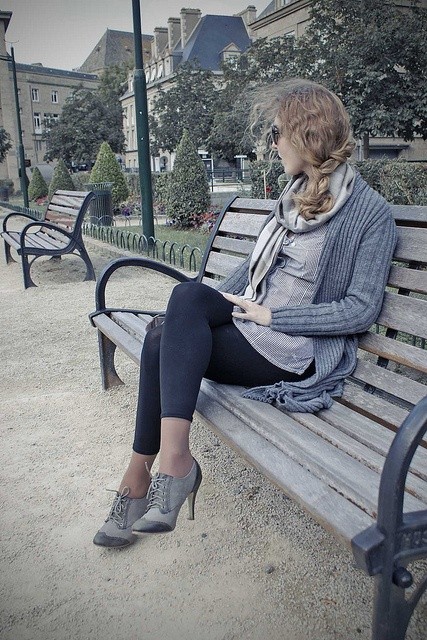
[83, 182, 114, 226]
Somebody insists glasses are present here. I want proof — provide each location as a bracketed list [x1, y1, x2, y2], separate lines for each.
[271, 123, 282, 145]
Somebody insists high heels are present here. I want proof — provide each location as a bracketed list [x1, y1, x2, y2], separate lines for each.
[131, 456, 203, 536]
[93, 478, 157, 549]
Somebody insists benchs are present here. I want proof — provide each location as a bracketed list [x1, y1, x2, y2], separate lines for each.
[0, 189, 96, 289]
[88, 192, 427, 640]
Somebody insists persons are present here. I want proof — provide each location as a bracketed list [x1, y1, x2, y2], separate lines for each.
[92, 81, 399, 551]
